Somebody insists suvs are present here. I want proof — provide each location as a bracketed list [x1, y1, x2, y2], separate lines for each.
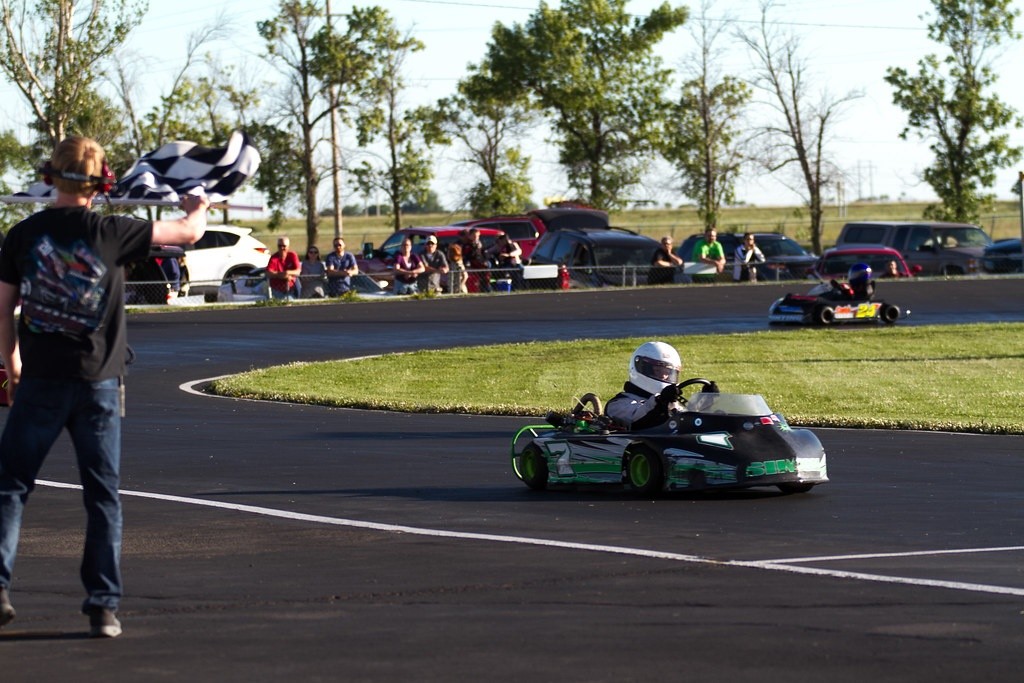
[357, 207, 1024, 290]
[183, 224, 272, 302]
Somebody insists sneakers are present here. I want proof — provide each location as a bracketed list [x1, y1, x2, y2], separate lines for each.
[0, 588, 15, 626]
[90, 608, 122, 638]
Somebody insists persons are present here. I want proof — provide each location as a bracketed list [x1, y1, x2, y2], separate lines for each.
[734, 233, 765, 283]
[691, 229, 726, 283]
[840, 263, 873, 302]
[393, 229, 522, 294]
[605, 341, 720, 430]
[0, 138, 210, 636]
[882, 260, 906, 278]
[266, 237, 358, 300]
[648, 237, 683, 284]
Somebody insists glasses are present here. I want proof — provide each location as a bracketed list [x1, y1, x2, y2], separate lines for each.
[277, 245, 288, 248]
[333, 243, 343, 248]
[308, 250, 318, 255]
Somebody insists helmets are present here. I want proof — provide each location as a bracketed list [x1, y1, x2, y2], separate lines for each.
[628, 341, 682, 397]
[848, 263, 871, 286]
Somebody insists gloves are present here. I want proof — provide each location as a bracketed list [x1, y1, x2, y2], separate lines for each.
[701, 381, 720, 394]
[660, 385, 682, 403]
[840, 283, 854, 294]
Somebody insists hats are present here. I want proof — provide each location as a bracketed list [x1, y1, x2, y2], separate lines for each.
[469, 228, 479, 235]
[426, 236, 437, 245]
